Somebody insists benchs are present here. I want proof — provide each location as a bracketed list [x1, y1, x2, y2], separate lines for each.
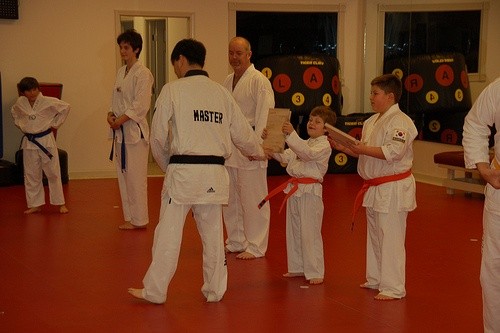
[434, 151, 494, 195]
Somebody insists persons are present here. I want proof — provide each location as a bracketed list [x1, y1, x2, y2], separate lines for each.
[328, 74, 418, 300]
[106, 31, 154, 231]
[261, 106, 336, 284]
[10, 77, 71, 214]
[128, 37, 275, 303]
[462, 76, 500, 333]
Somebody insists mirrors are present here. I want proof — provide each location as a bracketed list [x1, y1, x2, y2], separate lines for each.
[116, 9, 194, 164]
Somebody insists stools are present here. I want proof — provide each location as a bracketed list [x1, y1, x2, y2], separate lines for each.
[16, 148, 68, 184]
